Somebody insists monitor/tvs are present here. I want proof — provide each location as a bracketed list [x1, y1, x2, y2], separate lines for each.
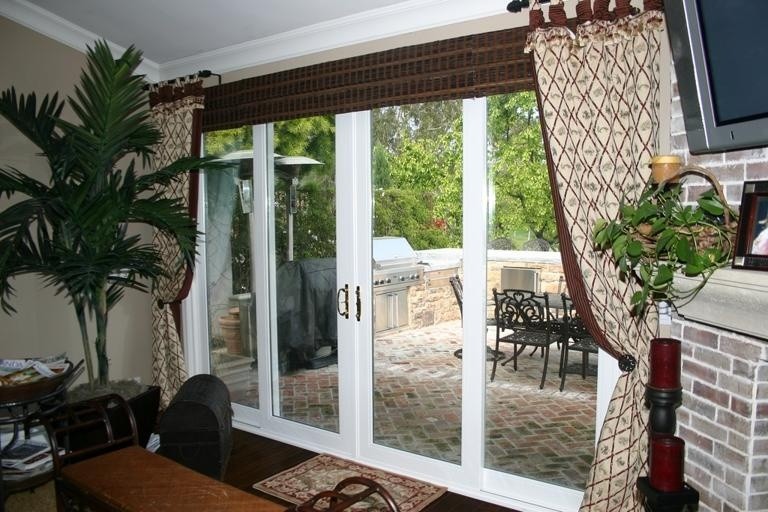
[661, 0, 768, 156]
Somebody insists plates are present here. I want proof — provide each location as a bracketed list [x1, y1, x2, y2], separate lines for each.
[0, 360, 74, 404]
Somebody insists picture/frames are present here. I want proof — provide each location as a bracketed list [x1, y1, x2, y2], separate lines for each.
[732, 180, 768, 274]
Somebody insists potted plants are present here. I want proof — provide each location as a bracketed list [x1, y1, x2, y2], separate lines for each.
[593, 167, 740, 318]
[1, 37, 241, 466]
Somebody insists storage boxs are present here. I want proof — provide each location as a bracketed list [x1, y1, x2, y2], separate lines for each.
[159, 374, 233, 483]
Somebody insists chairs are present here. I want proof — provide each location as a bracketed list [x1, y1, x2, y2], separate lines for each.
[556, 276, 571, 350]
[559, 293, 598, 391]
[450, 275, 506, 361]
[490, 288, 565, 389]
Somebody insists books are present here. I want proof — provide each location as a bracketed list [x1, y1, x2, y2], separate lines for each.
[0, 353, 70, 386]
[0, 439, 65, 470]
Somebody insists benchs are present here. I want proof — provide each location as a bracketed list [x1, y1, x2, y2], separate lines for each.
[42, 394, 399, 512]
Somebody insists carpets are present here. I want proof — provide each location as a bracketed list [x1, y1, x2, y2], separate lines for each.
[253, 453, 448, 512]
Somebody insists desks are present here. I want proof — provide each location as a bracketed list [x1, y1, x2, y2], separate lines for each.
[0, 358, 83, 499]
[492, 293, 577, 366]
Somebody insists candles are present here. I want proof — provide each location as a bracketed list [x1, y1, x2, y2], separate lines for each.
[649, 435, 685, 493]
[651, 339, 680, 388]
